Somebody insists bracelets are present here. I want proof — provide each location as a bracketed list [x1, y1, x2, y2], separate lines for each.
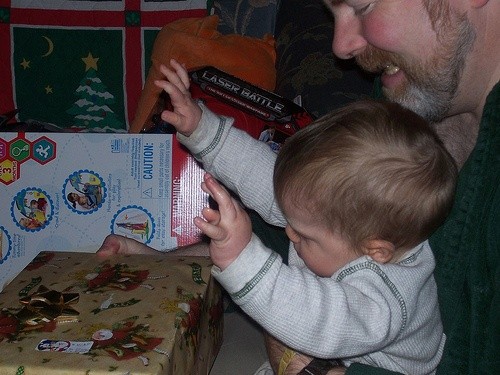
[278, 347, 296, 375]
[297, 357, 337, 375]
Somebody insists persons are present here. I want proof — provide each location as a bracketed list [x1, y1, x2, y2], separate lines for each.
[153, 58, 458, 375]
[95, 0, 499, 374]
